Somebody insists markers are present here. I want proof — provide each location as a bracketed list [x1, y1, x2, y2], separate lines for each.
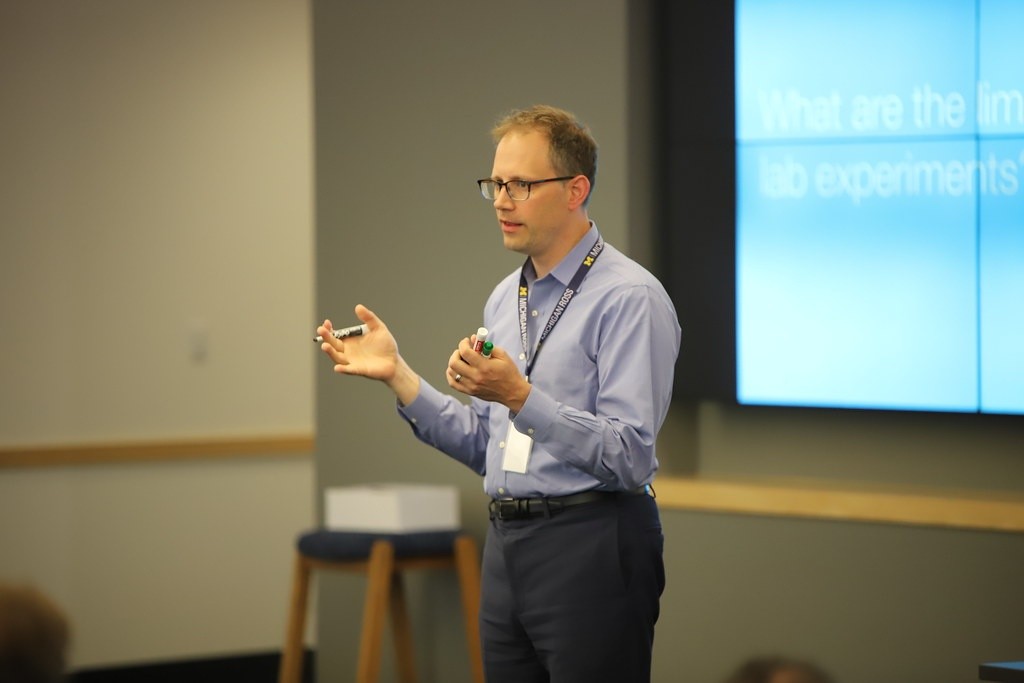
[481, 341, 494, 358]
[312, 321, 369, 341]
[472, 327, 488, 354]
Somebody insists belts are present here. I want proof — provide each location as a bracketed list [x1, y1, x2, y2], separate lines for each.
[492, 485, 651, 519]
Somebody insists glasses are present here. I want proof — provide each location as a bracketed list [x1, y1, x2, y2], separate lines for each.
[477, 175, 575, 201]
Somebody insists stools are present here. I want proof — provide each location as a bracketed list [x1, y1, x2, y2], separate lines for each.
[278, 528, 484, 683]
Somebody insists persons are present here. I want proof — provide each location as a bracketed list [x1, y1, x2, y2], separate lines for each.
[317, 106, 683, 682]
